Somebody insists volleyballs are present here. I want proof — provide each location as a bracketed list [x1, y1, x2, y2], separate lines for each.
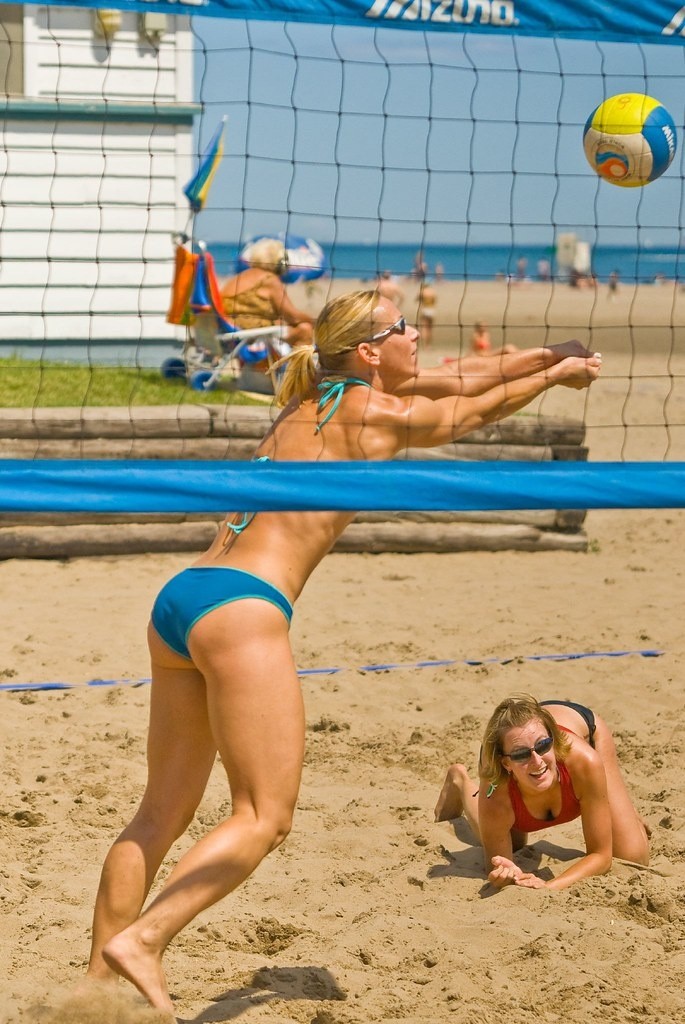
[581, 91, 679, 188]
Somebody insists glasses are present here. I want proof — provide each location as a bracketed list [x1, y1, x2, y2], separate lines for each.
[498, 737, 554, 762]
[336, 317, 406, 356]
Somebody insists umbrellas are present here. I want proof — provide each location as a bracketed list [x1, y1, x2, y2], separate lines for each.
[182, 114, 228, 234]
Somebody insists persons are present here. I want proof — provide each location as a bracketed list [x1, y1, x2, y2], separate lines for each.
[219, 237, 317, 373]
[376, 243, 443, 349]
[434, 691, 652, 893]
[464, 319, 521, 358]
[87, 287, 605, 1024]
[495, 254, 670, 294]
